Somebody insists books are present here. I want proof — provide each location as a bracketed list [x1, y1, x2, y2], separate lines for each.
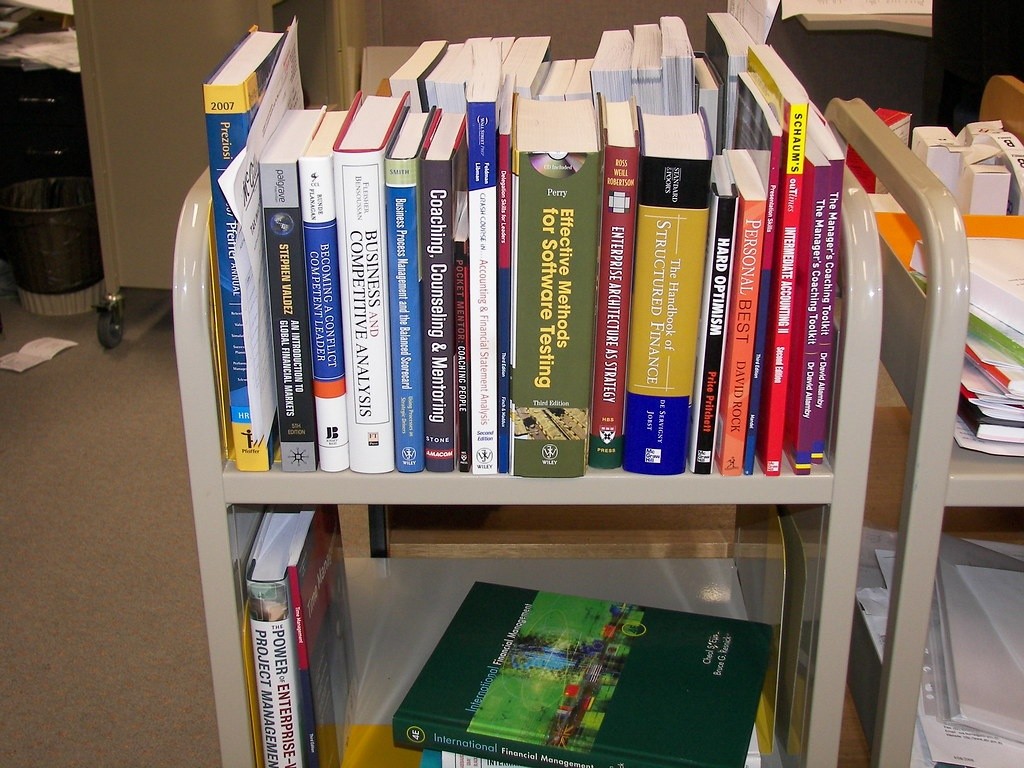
[908, 236, 1024, 457]
[795, 11, 932, 37]
[199, 11, 845, 479]
[241, 507, 774, 768]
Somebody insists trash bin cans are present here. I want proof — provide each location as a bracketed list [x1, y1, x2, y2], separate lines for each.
[1, 175, 104, 317]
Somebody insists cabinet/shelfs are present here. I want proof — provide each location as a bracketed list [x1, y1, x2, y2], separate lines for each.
[169, 97, 1024, 768]
